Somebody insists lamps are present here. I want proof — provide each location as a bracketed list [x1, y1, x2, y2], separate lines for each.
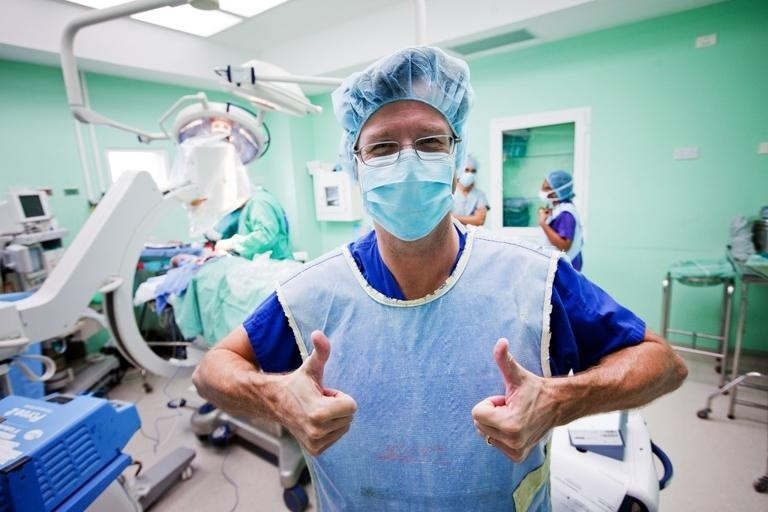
[161, 59, 324, 167]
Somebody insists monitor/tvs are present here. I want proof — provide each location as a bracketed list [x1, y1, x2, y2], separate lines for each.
[13, 191, 52, 222]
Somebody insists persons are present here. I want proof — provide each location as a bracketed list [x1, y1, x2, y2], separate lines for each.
[192, 44, 689, 511]
[538, 170, 584, 272]
[201, 185, 293, 265]
[203, 207, 244, 242]
[169, 250, 198, 271]
[449, 151, 491, 226]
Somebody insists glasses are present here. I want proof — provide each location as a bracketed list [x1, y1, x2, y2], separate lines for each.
[352, 134, 464, 169]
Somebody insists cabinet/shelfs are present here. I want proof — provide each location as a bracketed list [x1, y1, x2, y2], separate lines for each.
[661, 260, 733, 396]
[716, 247, 768, 420]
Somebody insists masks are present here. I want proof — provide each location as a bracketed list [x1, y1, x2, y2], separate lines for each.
[538, 189, 555, 210]
[350, 148, 460, 242]
[458, 172, 476, 187]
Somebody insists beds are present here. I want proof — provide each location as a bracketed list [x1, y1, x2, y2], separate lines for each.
[136, 274, 660, 512]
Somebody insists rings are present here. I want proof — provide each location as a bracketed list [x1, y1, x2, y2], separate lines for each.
[486, 435, 492, 446]
